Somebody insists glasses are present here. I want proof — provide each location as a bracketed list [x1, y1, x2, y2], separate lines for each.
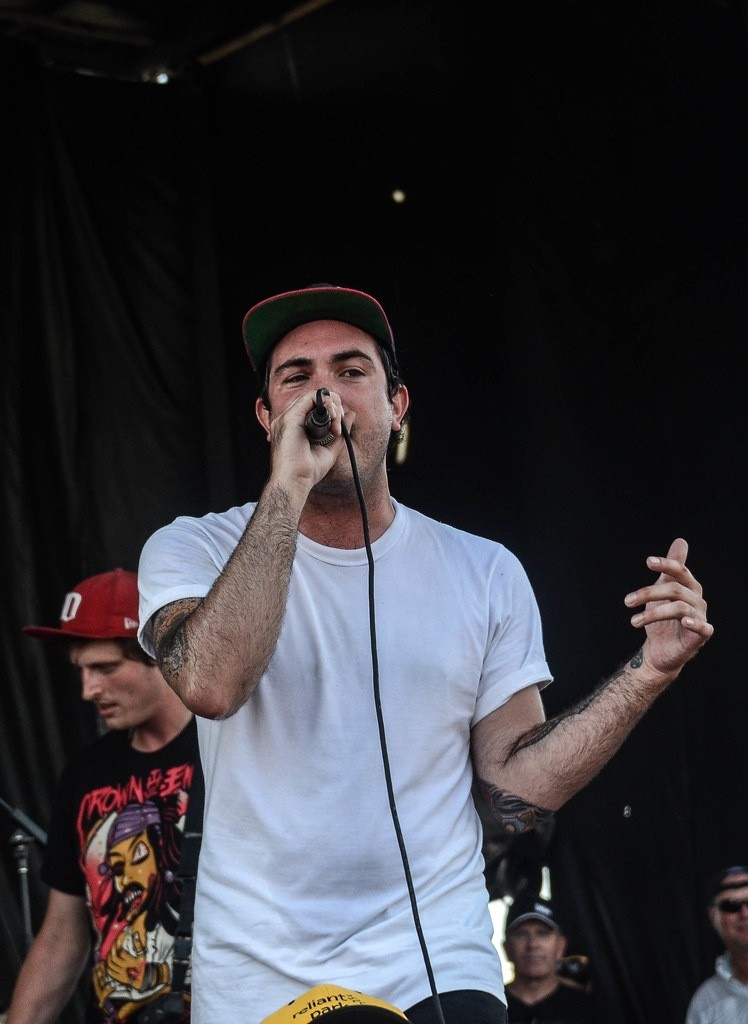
[709, 900, 747, 912]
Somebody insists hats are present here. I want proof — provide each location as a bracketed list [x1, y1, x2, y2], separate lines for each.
[240, 282, 397, 385]
[21, 567, 139, 639]
[507, 898, 560, 931]
[701, 861, 748, 908]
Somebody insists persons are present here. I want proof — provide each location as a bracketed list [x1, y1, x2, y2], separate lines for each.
[0, 567, 204, 1024]
[685, 866, 748, 1024]
[503, 896, 595, 1023]
[136, 286, 714, 1024]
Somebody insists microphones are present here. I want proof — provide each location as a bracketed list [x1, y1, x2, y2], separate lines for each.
[304, 407, 335, 447]
